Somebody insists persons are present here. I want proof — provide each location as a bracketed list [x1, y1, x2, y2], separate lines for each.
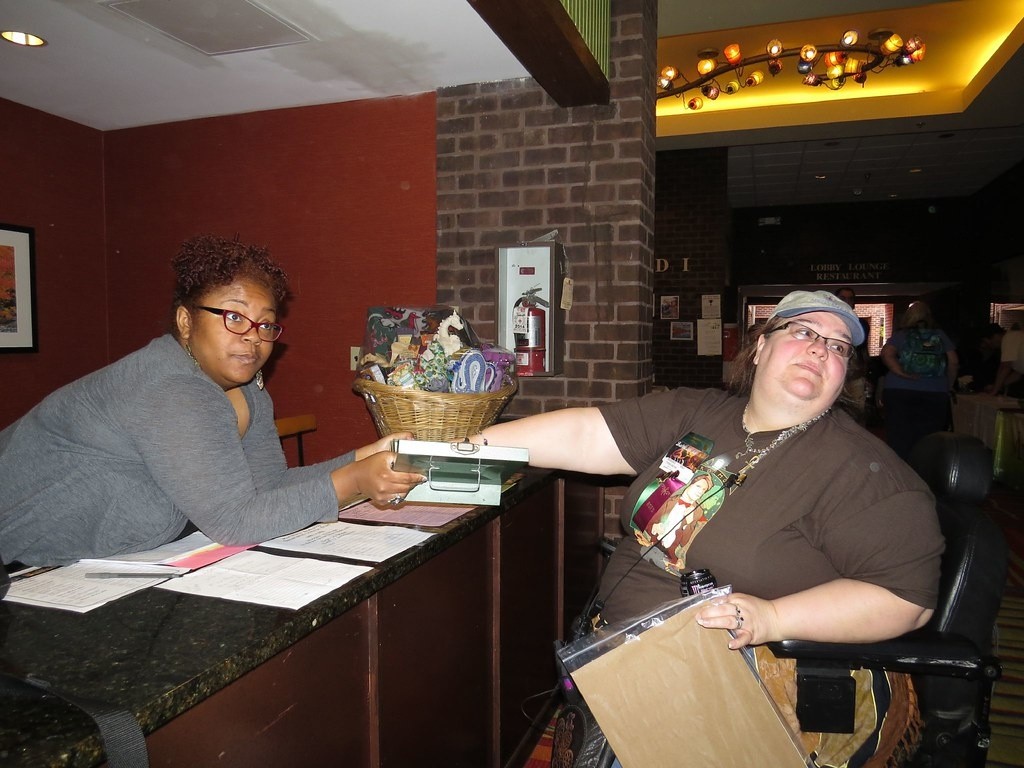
[450, 290, 948, 768]
[0, 234, 428, 571]
[835, 286, 1024, 459]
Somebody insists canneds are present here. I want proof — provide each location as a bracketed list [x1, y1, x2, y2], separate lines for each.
[680, 568, 717, 598]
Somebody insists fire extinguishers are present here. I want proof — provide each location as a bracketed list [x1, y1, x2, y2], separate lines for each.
[510, 288, 550, 376]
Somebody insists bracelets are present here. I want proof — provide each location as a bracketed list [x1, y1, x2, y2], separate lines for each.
[474, 431, 488, 445]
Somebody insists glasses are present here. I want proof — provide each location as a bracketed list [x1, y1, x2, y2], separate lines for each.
[769, 321, 855, 356]
[196, 305, 283, 343]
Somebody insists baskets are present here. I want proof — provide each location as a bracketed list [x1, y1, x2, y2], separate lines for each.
[354, 363, 517, 441]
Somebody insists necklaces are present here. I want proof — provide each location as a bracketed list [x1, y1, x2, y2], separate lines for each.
[733, 402, 830, 459]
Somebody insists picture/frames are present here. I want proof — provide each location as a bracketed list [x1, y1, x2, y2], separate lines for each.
[660, 295, 680, 320]
[0, 223, 40, 354]
[670, 321, 694, 342]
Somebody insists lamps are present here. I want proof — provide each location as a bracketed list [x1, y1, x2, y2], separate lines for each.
[656, 30, 926, 111]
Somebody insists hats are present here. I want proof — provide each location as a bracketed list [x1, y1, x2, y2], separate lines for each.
[767, 290, 865, 345]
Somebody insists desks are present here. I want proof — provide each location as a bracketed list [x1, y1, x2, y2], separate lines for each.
[949, 394, 1024, 451]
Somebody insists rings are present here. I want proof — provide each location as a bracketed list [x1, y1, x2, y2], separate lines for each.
[734, 616, 744, 629]
[733, 604, 740, 616]
[389, 493, 405, 506]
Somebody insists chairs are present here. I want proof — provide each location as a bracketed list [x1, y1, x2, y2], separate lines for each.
[766, 431, 1011, 768]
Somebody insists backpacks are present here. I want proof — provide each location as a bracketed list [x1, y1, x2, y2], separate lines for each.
[896, 320, 948, 383]
